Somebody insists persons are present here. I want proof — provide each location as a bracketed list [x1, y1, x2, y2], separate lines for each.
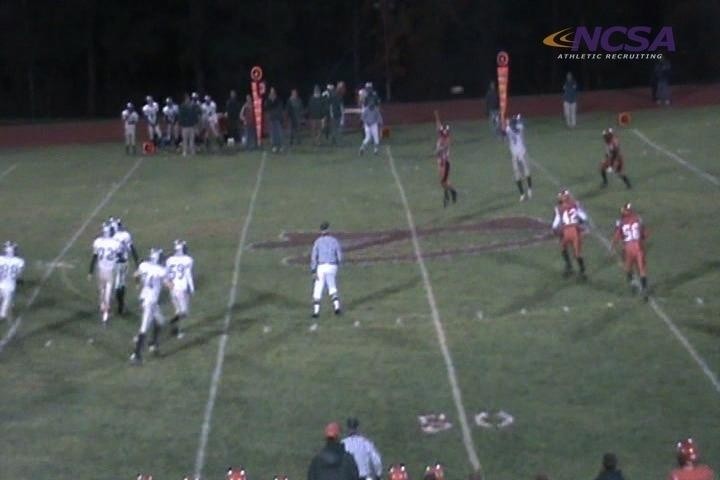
[307, 423, 360, 480]
[120, 78, 389, 158]
[500, 111, 533, 202]
[559, 71, 577, 129]
[485, 80, 499, 131]
[86, 219, 125, 325]
[608, 202, 651, 292]
[599, 128, 631, 191]
[165, 238, 195, 336]
[550, 187, 592, 279]
[593, 454, 626, 480]
[669, 435, 713, 479]
[129, 247, 175, 357]
[341, 417, 382, 480]
[0, 239, 27, 321]
[309, 221, 343, 317]
[431, 109, 456, 206]
[136, 461, 447, 480]
[102, 217, 136, 315]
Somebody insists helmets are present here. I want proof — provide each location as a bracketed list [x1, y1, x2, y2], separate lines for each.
[388, 463, 408, 480]
[225, 467, 247, 480]
[99, 217, 186, 264]
[556, 190, 569, 203]
[619, 202, 634, 216]
[601, 128, 612, 140]
[675, 439, 698, 465]
[422, 464, 444, 480]
[5, 241, 18, 256]
